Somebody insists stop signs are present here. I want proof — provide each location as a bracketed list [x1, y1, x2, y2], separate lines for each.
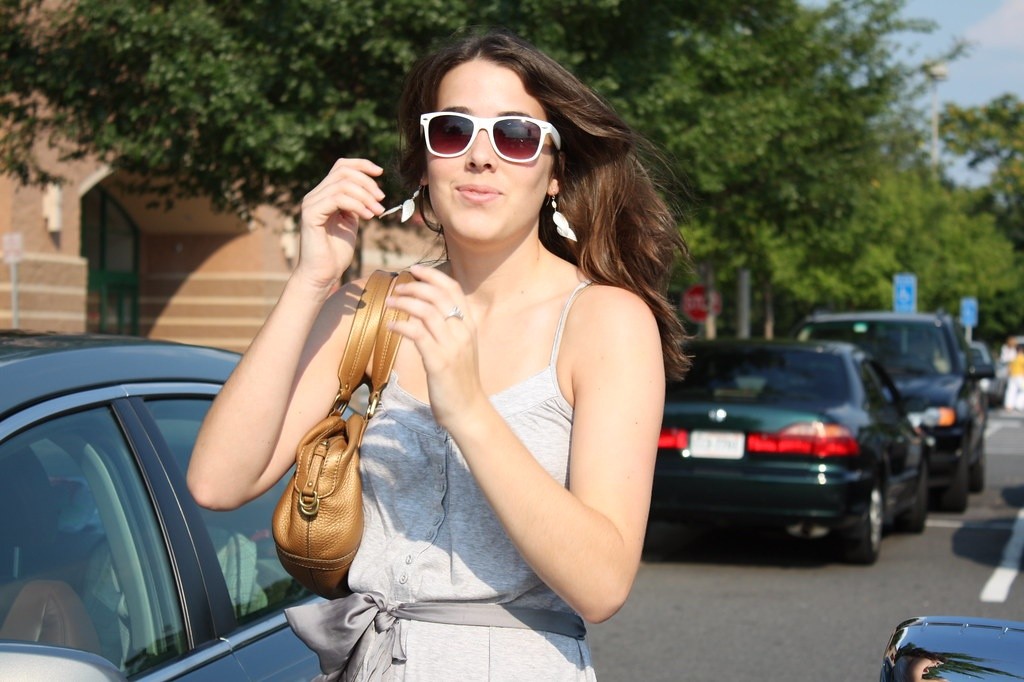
[684, 285, 722, 321]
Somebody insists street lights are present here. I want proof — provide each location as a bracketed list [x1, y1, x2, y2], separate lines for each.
[922, 57, 948, 187]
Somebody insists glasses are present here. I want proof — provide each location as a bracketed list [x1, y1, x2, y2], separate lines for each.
[419, 111, 561, 163]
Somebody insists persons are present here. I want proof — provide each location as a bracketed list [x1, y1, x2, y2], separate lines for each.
[999, 336, 1024, 413]
[184, 32, 701, 682]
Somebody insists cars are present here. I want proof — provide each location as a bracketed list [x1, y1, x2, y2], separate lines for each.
[643, 337, 932, 564]
[969, 341, 1008, 407]
[0, 324, 363, 682]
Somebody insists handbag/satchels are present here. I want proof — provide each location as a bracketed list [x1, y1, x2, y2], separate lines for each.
[271, 269, 422, 601]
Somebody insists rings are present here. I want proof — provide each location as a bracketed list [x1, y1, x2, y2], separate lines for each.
[444, 304, 464, 322]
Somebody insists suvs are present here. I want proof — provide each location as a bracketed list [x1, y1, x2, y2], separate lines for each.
[790, 308, 995, 514]
